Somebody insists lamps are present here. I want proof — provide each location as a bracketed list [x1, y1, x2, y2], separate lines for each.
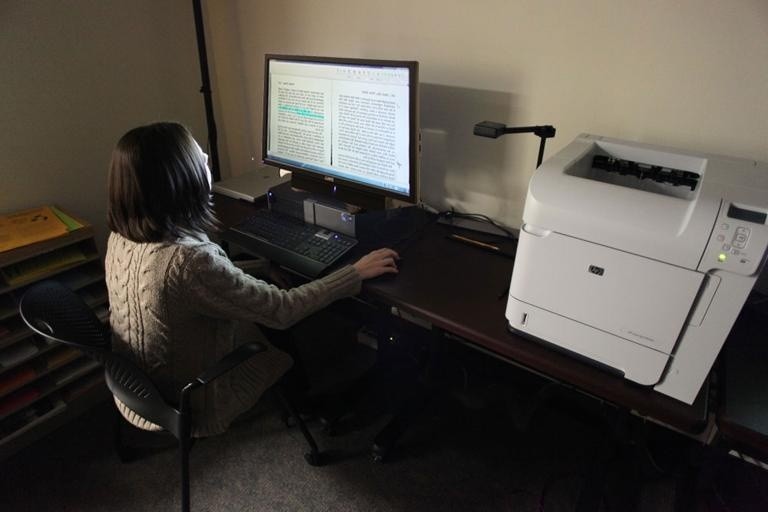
[473, 119, 557, 260]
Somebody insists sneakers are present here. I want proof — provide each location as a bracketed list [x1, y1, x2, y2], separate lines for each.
[286, 410, 357, 434]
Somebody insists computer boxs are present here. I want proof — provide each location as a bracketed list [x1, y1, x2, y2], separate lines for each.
[263, 180, 427, 246]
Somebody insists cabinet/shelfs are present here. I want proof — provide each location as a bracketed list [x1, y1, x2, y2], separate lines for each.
[0, 202, 109, 468]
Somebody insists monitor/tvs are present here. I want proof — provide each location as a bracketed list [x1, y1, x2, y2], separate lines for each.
[262, 54, 420, 211]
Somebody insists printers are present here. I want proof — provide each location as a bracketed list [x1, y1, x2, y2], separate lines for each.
[505, 132, 768, 405]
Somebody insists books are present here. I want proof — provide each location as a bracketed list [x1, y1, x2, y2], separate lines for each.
[0, 204, 111, 448]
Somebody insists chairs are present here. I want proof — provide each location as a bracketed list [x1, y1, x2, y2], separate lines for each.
[19, 282, 337, 512]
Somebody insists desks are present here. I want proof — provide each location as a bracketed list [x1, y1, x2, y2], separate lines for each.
[196, 170, 768, 512]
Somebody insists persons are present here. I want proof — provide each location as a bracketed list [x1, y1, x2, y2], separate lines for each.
[104, 122, 399, 440]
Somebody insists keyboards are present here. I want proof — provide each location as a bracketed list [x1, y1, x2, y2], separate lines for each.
[229, 209, 360, 278]
